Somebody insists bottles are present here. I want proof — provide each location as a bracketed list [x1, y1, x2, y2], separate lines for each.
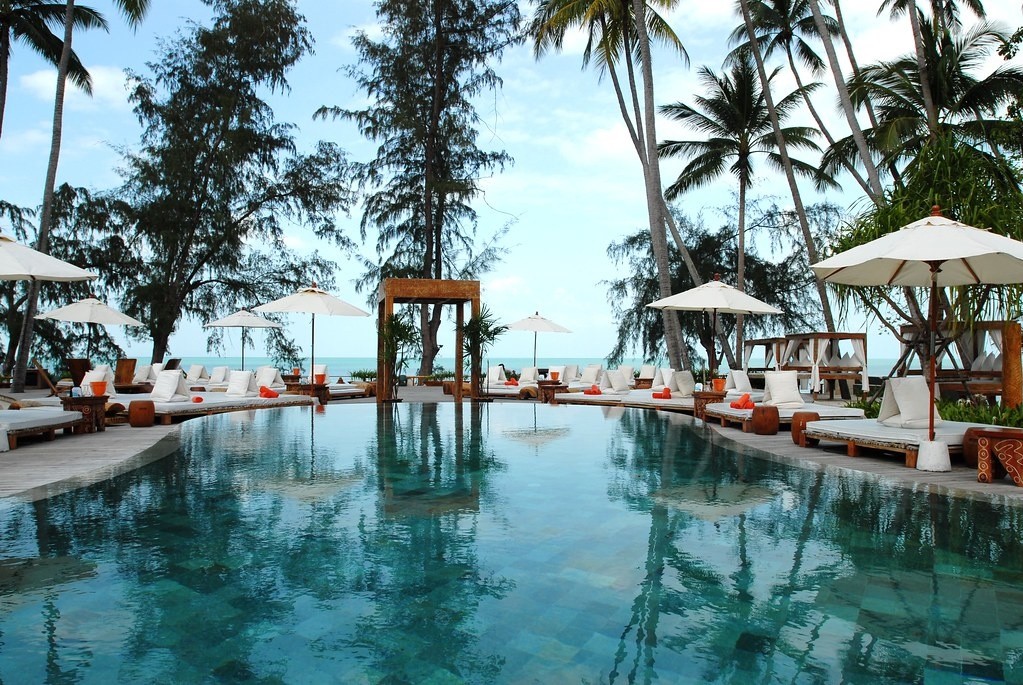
[72, 387, 82, 397]
[82, 385, 92, 397]
[293, 368, 299, 375]
[695, 382, 703, 391]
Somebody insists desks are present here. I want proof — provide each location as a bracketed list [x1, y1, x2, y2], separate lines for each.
[60, 396, 110, 433]
[693, 390, 728, 419]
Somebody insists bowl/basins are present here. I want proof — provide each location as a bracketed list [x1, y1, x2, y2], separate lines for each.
[551, 372, 559, 380]
[315, 374, 326, 384]
[90, 382, 107, 396]
[712, 379, 726, 391]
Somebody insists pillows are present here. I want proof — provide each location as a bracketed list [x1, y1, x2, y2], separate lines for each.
[970, 351, 1004, 381]
[78, 363, 332, 402]
[484, 352, 944, 426]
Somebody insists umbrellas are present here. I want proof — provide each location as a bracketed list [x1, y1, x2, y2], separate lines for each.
[0, 227, 100, 282]
[809, 205, 1023, 441]
[251, 282, 372, 397]
[505, 311, 574, 367]
[650, 428, 783, 532]
[645, 273, 785, 391]
[258, 406, 364, 510]
[202, 307, 282, 371]
[501, 403, 569, 456]
[812, 569, 1023, 669]
[32, 294, 145, 359]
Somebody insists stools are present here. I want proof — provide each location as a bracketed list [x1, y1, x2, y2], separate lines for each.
[963, 426, 986, 465]
[127, 399, 156, 428]
[751, 405, 779, 436]
[789, 412, 821, 448]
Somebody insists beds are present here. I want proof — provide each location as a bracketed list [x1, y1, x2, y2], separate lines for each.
[0, 381, 365, 453]
[486, 374, 995, 469]
